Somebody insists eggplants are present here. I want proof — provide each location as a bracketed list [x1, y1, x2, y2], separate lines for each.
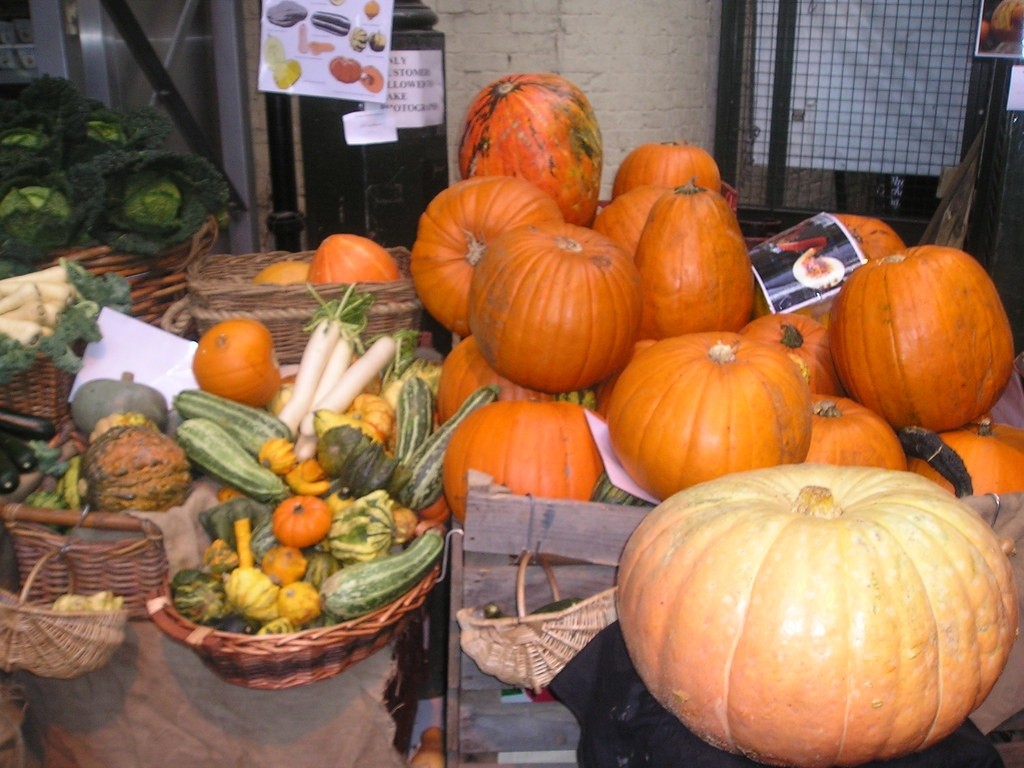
[1, 412, 54, 495]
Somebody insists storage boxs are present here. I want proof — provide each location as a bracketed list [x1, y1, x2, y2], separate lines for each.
[437, 467, 655, 765]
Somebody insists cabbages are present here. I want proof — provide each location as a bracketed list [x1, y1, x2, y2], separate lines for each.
[0, 72, 233, 278]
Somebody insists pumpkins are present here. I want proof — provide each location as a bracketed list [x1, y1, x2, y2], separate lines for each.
[72, 74, 1023, 767]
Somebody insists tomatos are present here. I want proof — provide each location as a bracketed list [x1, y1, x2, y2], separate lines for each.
[329, 56, 384, 92]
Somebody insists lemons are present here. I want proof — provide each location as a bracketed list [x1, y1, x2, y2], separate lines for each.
[264, 34, 300, 89]
